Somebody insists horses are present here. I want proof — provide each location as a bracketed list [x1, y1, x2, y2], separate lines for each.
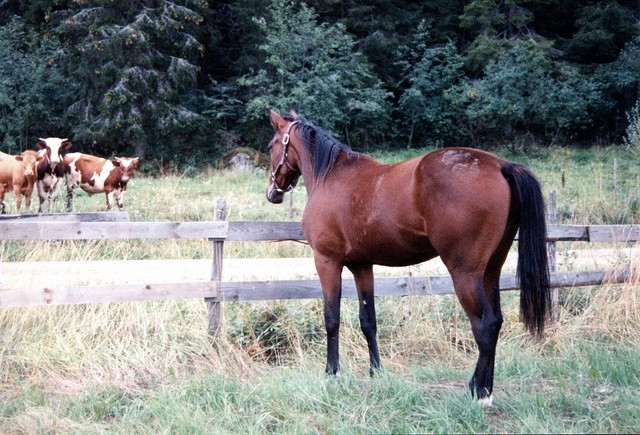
[265, 107, 556, 409]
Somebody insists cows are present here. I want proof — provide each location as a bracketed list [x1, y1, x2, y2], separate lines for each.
[0, 150, 43, 215]
[36, 138, 72, 215]
[63, 152, 140, 211]
